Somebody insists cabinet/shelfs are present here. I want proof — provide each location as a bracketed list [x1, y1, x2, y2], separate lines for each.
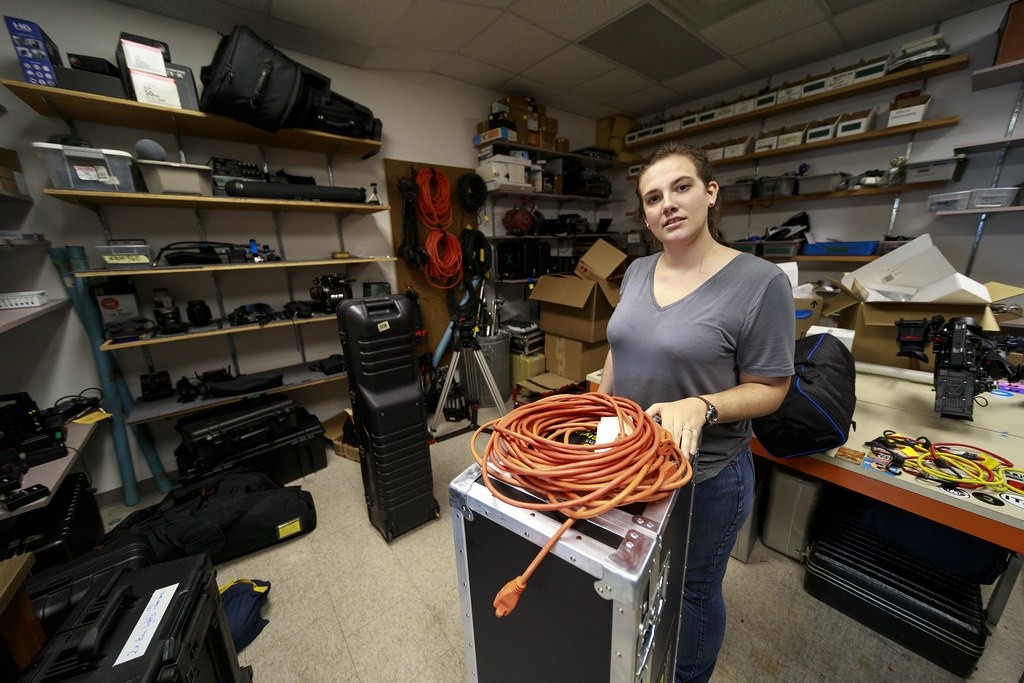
[625, 53, 968, 264]
[935, 57, 1023, 214]
[477, 141, 625, 283]
[0, 76, 397, 518]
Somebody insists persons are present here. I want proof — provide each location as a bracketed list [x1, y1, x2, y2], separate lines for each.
[596, 141, 795, 683]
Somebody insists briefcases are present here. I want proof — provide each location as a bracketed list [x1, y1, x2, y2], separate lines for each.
[17, 553, 254, 683]
[23, 533, 153, 637]
[795, 518, 995, 679]
[174, 394, 298, 458]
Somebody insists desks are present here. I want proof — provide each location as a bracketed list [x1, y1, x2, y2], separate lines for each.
[747, 369, 1024, 626]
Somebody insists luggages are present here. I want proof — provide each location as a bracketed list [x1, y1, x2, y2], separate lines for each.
[173, 408, 327, 486]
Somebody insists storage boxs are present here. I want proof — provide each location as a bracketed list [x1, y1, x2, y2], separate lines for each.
[595, 0, 1023, 373]
[449, 414, 699, 683]
[0, 147, 29, 195]
[801, 514, 992, 680]
[504, 236, 631, 407]
[30, 141, 134, 192]
[320, 406, 358, 462]
[472, 92, 569, 195]
[135, 159, 212, 196]
[174, 406, 329, 484]
[94, 243, 154, 270]
[3, 14, 200, 109]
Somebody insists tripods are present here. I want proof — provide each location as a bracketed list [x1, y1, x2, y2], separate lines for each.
[429, 324, 509, 435]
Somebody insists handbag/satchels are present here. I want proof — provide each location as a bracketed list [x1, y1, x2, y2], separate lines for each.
[214, 576, 272, 656]
[194, 24, 375, 137]
[751, 333, 857, 460]
[96, 471, 317, 566]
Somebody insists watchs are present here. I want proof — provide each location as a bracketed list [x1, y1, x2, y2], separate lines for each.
[695, 396, 718, 427]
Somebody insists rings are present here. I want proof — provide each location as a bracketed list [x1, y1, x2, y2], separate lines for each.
[682, 427, 693, 432]
